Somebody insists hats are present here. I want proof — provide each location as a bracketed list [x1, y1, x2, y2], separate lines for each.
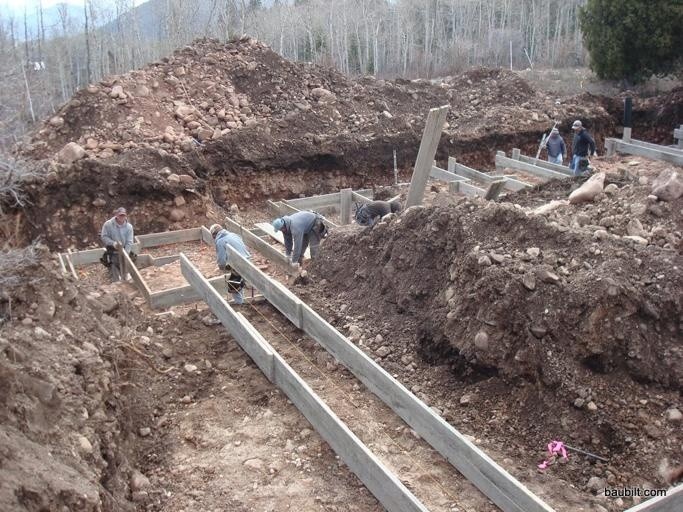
[393, 202, 402, 212]
[571, 121, 582, 129]
[552, 128, 559, 135]
[273, 219, 284, 232]
[116, 207, 126, 216]
[210, 224, 221, 238]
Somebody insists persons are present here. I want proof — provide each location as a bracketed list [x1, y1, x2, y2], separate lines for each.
[273, 210, 328, 265]
[100, 207, 133, 282]
[542, 127, 566, 165]
[209, 223, 250, 305]
[568, 119, 595, 176]
[363, 199, 402, 227]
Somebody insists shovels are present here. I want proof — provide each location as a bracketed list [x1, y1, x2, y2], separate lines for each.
[544, 118, 563, 145]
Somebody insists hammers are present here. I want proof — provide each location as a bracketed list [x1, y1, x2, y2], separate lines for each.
[225, 280, 244, 288]
